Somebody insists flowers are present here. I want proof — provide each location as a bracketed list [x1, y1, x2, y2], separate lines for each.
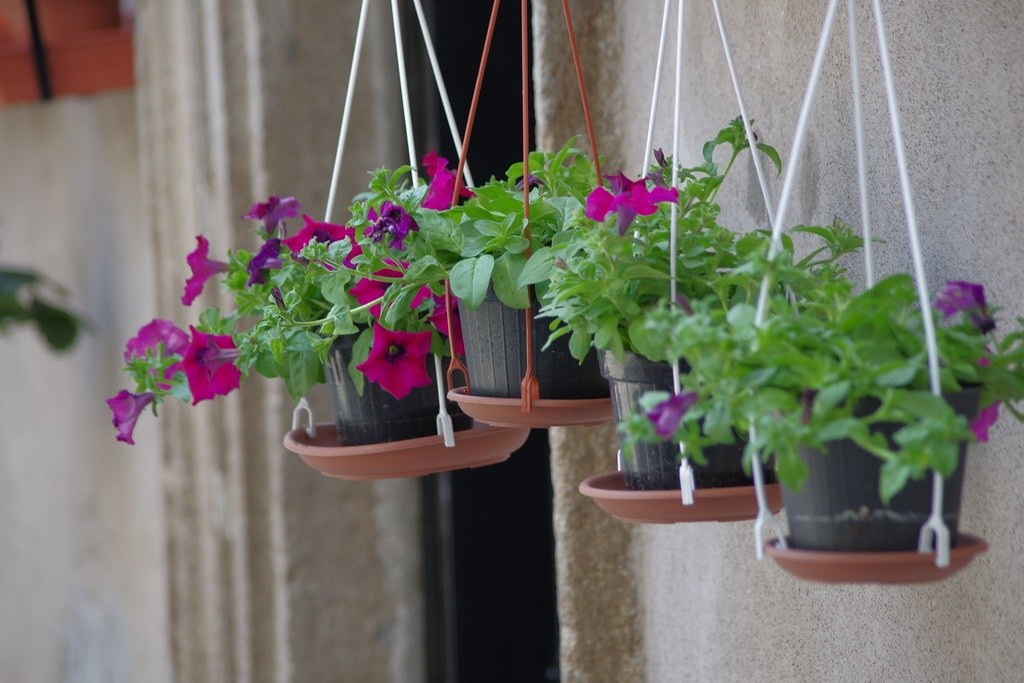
[103, 113, 1023, 457]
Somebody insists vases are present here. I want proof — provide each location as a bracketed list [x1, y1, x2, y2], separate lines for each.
[328, 321, 473, 449]
[449, 274, 610, 399]
[594, 338, 778, 490]
[771, 379, 983, 552]
